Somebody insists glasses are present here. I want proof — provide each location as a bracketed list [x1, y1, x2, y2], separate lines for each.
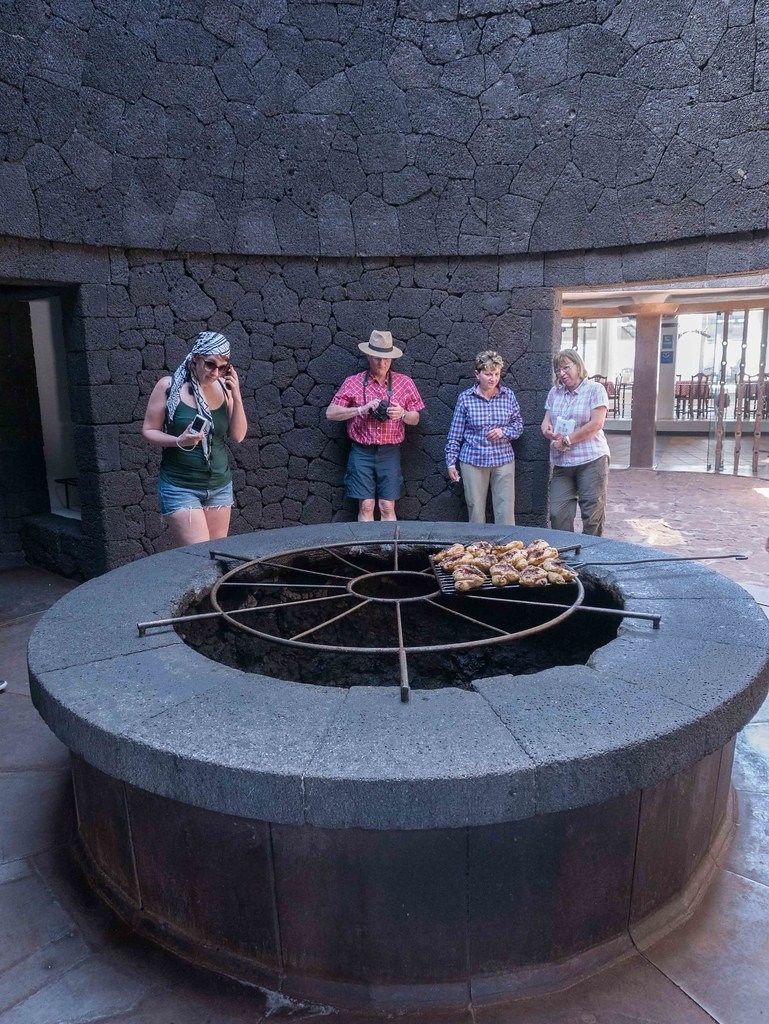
[554, 362, 575, 374]
[201, 357, 230, 374]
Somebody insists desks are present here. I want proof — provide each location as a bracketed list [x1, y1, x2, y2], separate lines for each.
[735, 379, 768, 419]
[591, 378, 615, 418]
[674, 380, 713, 420]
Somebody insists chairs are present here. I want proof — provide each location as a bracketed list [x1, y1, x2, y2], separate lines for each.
[585, 372, 769, 420]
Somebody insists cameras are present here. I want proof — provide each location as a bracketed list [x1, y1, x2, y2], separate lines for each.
[368, 400, 391, 422]
[189, 415, 208, 435]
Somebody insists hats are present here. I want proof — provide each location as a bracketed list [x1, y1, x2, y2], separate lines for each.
[358, 330, 403, 358]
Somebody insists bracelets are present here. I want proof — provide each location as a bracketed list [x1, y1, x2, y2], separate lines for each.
[566, 435, 571, 444]
[562, 437, 572, 447]
[400, 410, 406, 421]
[358, 407, 364, 418]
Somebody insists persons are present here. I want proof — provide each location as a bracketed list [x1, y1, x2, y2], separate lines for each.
[142, 332, 247, 546]
[444, 352, 523, 525]
[541, 349, 611, 537]
[326, 330, 425, 520]
